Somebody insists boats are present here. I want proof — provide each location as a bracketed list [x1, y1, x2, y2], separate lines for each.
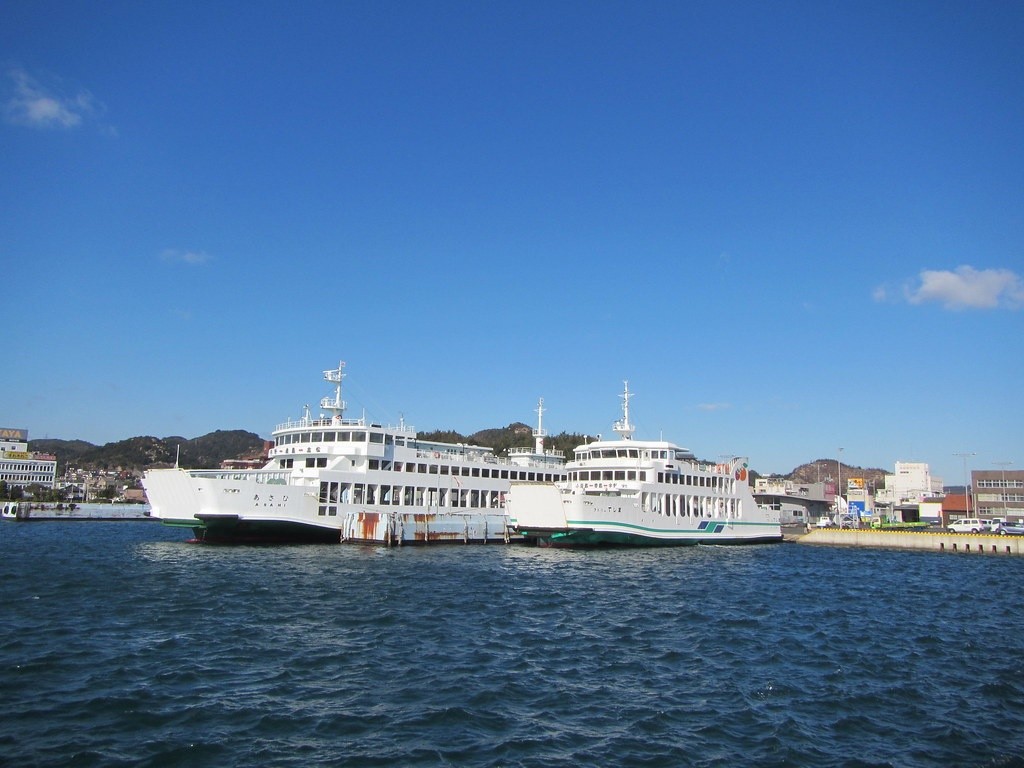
[506, 378, 783, 548]
[139, 359, 569, 549]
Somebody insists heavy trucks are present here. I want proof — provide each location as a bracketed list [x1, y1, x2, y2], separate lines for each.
[870, 516, 932, 532]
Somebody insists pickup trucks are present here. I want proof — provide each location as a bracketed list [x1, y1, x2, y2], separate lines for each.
[816, 517, 833, 528]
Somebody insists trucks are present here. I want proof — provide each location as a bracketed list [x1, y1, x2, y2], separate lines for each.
[989, 517, 1007, 532]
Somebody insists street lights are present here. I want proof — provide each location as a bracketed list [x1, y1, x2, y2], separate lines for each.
[837, 447, 844, 530]
[952, 452, 978, 520]
[991, 461, 1014, 525]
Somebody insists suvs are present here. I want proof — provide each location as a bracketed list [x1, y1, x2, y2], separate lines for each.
[999, 522, 1024, 535]
[946, 518, 984, 534]
[979, 518, 993, 531]
